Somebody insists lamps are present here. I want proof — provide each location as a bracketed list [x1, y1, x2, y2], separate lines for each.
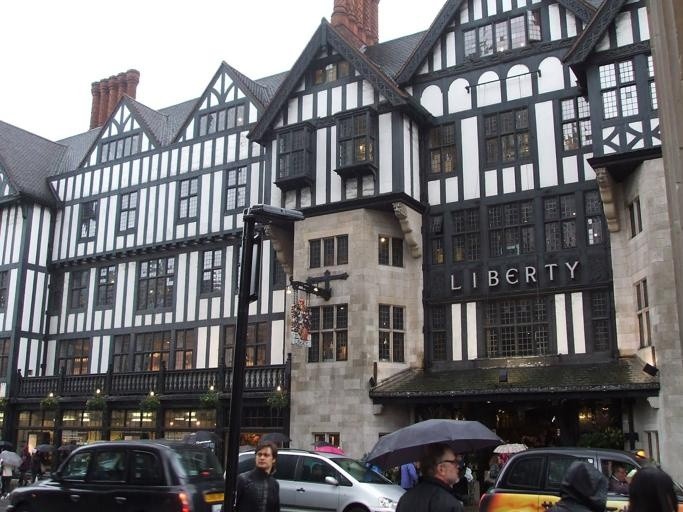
[368, 374, 377, 387]
[641, 361, 659, 377]
[496, 368, 508, 383]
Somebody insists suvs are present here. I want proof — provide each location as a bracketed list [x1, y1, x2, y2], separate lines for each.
[6, 440, 225, 510]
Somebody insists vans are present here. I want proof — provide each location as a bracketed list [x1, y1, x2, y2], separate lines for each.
[222, 449, 407, 511]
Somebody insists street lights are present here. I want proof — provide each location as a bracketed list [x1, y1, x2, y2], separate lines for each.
[221, 203, 304, 511]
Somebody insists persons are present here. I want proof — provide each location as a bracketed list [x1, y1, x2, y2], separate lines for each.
[0, 441, 45, 499]
[546, 460, 609, 511]
[628, 465, 678, 511]
[365, 447, 510, 500]
[235, 441, 281, 512]
[396, 443, 464, 511]
[608, 461, 630, 494]
[627, 450, 647, 478]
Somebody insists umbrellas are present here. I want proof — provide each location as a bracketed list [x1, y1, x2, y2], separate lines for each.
[318, 445, 343, 455]
[59, 444, 77, 451]
[37, 443, 56, 453]
[259, 434, 294, 445]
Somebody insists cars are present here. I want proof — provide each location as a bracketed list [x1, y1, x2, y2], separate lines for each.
[476, 447, 683, 512]
